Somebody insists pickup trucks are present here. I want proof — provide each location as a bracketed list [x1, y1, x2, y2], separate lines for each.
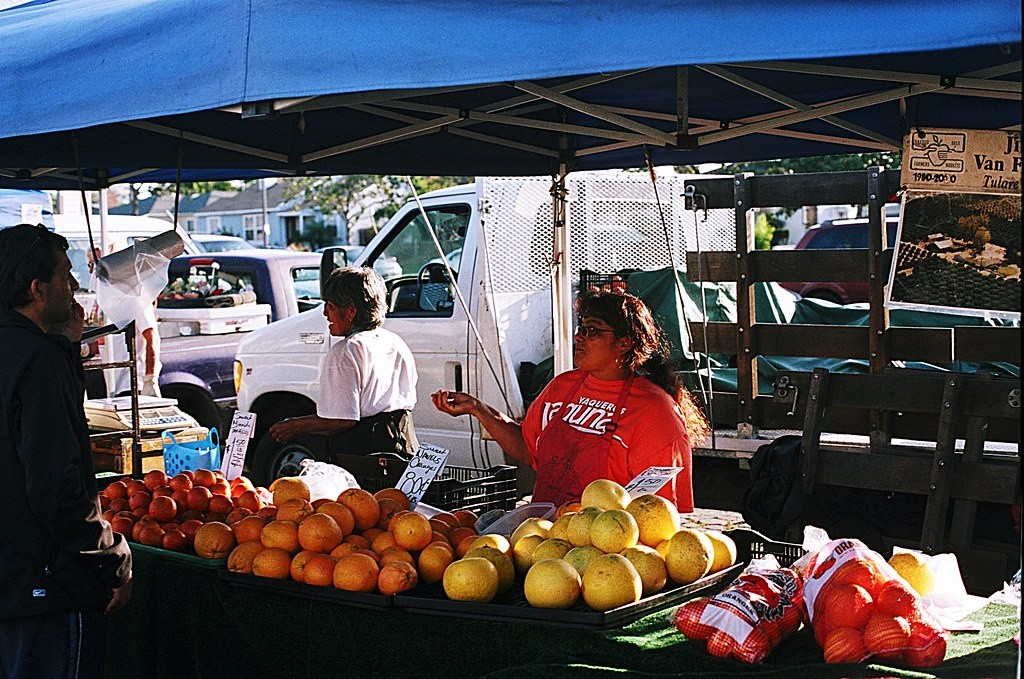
[84, 248, 326, 442]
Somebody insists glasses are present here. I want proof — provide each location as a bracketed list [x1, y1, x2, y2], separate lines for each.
[574, 324, 616, 337]
[14, 223, 50, 271]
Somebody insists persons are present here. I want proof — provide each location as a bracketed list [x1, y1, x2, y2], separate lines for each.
[431, 292, 696, 516]
[271, 267, 420, 458]
[87, 238, 163, 399]
[0, 225, 132, 678]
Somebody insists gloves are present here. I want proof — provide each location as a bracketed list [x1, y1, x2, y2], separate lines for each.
[141, 373, 161, 397]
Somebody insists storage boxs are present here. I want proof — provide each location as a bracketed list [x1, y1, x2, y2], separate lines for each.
[578, 269, 630, 293]
[728, 529, 806, 569]
[157, 304, 272, 334]
[325, 449, 518, 518]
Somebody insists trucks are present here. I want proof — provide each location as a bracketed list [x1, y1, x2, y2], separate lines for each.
[229, 176, 1021, 580]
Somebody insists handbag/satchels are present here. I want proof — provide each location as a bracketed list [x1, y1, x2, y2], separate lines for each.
[76, 531, 132, 622]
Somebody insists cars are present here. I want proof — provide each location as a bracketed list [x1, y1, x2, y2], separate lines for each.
[428, 246, 460, 274]
[50, 214, 271, 338]
[296, 245, 402, 280]
[187, 233, 257, 253]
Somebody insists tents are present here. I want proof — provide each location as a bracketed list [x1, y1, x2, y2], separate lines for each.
[1, 0, 1024, 379]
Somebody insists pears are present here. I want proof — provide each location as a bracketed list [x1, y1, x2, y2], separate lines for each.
[442, 479, 737, 611]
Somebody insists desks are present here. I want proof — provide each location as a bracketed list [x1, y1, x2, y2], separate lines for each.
[78, 542, 1019, 679]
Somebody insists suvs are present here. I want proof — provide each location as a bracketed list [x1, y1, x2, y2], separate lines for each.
[780, 217, 898, 304]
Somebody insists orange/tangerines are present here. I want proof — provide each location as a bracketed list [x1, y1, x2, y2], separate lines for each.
[674, 540, 946, 669]
[193, 480, 585, 595]
[93, 468, 273, 553]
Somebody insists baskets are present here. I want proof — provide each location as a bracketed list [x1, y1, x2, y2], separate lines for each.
[189, 426, 221, 472]
